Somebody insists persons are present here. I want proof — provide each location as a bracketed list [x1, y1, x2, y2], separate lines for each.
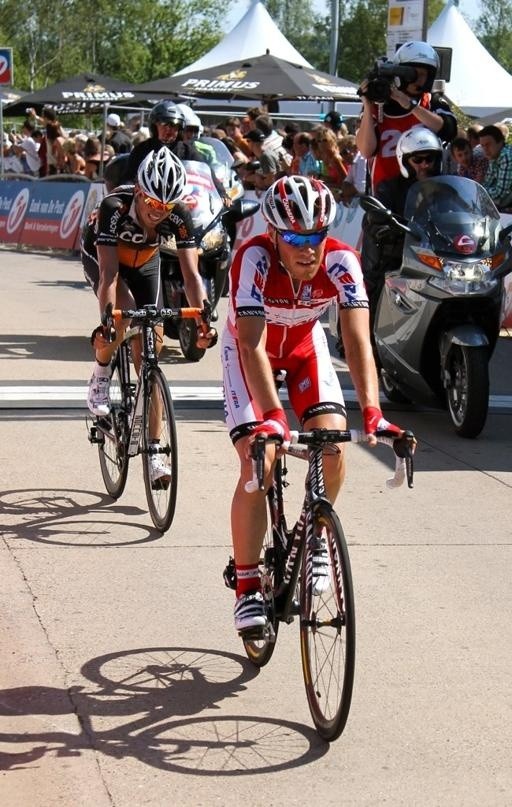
[354, 39, 458, 194]
[443, 122, 511, 214]
[180, 101, 366, 208]
[81, 144, 218, 480]
[367, 126, 479, 270]
[2, 107, 151, 180]
[107, 102, 233, 208]
[220, 175, 418, 632]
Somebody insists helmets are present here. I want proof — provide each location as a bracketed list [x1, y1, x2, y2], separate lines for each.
[394, 42, 440, 70]
[396, 128, 443, 178]
[138, 146, 186, 204]
[149, 100, 204, 141]
[261, 174, 337, 232]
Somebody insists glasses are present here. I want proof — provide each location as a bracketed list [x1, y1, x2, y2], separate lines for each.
[411, 155, 437, 164]
[143, 193, 176, 211]
[275, 227, 328, 245]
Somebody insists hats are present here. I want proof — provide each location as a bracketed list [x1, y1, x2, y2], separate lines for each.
[324, 112, 343, 124]
[107, 114, 121, 127]
[244, 129, 265, 142]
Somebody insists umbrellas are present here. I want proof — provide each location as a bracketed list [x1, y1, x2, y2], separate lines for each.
[112, 50, 362, 109]
[1, 71, 189, 132]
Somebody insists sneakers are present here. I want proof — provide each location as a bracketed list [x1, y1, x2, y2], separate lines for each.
[233, 588, 267, 629]
[88, 371, 112, 416]
[149, 443, 171, 481]
[312, 543, 332, 596]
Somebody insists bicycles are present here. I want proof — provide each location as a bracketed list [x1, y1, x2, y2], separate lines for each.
[88, 292, 218, 530]
[223, 421, 420, 740]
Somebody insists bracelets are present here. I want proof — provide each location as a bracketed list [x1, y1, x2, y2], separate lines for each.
[406, 99, 417, 114]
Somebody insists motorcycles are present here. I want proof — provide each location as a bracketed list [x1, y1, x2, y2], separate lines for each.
[195, 134, 263, 206]
[355, 176, 511, 440]
[102, 150, 259, 364]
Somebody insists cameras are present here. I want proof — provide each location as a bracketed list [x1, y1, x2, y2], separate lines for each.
[26, 108, 33, 114]
